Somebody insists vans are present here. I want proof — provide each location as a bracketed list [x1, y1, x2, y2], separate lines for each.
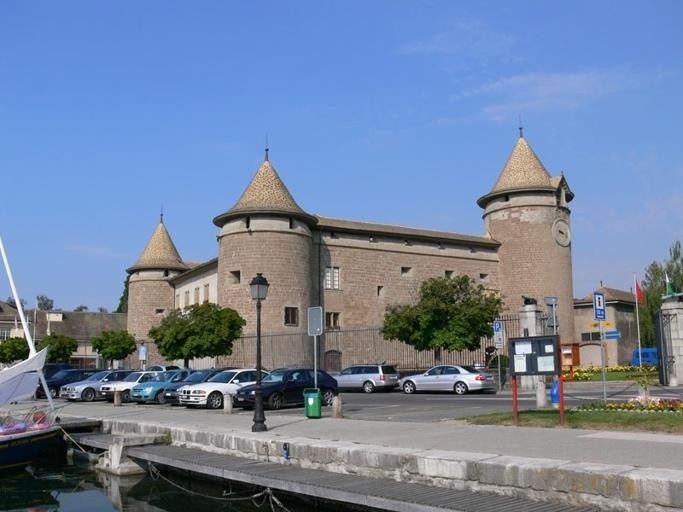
[333, 363, 400, 393]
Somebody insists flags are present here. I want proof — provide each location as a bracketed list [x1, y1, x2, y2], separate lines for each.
[665, 274, 674, 296]
[634, 278, 645, 304]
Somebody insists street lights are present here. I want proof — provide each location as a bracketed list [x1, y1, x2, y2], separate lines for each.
[248, 272, 269, 432]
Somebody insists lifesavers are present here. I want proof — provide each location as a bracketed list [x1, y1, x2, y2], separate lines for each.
[0, 422, 26, 435]
[28, 423, 49, 429]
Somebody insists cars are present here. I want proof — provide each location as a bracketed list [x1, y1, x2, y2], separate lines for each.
[35, 363, 214, 404]
[232, 367, 338, 409]
[398, 364, 494, 394]
[176, 367, 269, 409]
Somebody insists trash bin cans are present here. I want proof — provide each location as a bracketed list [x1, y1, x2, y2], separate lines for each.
[551, 382, 560, 408]
[303, 388, 320, 418]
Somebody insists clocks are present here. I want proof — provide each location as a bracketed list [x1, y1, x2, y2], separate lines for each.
[551, 218, 571, 248]
